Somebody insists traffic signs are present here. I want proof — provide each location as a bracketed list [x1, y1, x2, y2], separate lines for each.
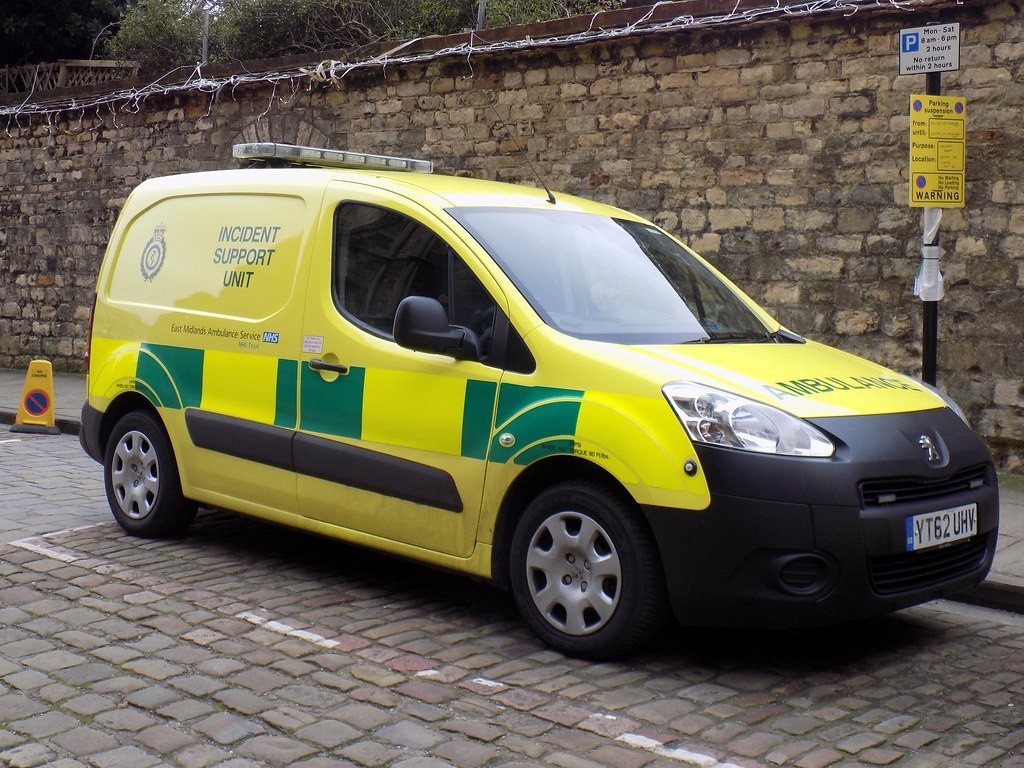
[898, 22, 960, 76]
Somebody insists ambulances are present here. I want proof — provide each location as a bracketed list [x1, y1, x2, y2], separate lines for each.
[74, 138, 1001, 665]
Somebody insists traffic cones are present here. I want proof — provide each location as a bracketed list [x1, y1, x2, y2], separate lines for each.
[10, 360, 62, 437]
